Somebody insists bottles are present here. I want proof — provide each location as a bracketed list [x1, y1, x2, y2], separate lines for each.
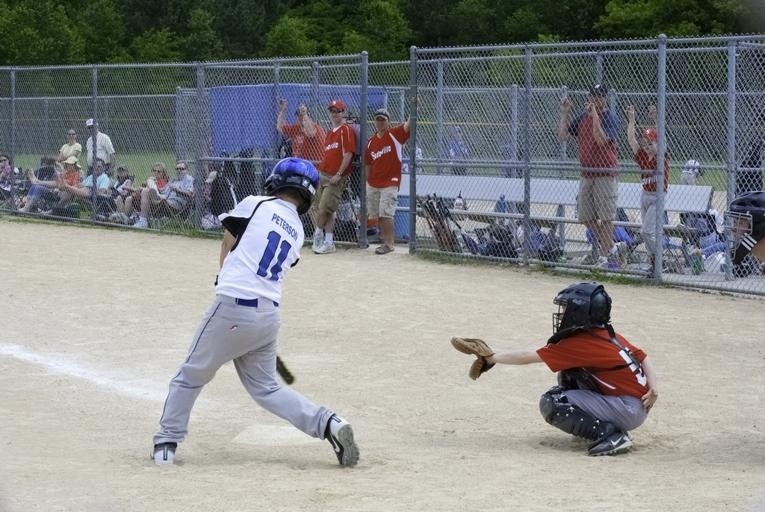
[588, 83, 609, 97]
[373, 107, 392, 122]
[641, 128, 658, 143]
[84, 118, 98, 129]
[326, 100, 345, 111]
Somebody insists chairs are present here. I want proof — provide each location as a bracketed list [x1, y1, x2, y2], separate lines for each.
[375, 243, 395, 256]
[323, 413, 361, 468]
[311, 235, 338, 255]
[148, 443, 178, 468]
[584, 432, 634, 459]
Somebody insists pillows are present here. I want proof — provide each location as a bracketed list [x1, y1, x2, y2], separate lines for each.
[263, 155, 319, 215]
[724, 187, 765, 265]
[547, 280, 616, 340]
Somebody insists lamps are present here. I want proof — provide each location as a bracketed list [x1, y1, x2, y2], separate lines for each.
[491, 199, 728, 273]
[0, 166, 197, 233]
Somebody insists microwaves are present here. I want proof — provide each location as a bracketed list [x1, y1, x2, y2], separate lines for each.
[415, 193, 478, 255]
[276, 357, 295, 383]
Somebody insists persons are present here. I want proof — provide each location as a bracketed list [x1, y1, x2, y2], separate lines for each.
[448, 127, 469, 172]
[58, 128, 81, 164]
[450, 281, 659, 458]
[340, 107, 361, 201]
[275, 97, 325, 241]
[203, 170, 238, 231]
[312, 100, 358, 255]
[145, 158, 361, 468]
[84, 117, 116, 173]
[557, 83, 623, 271]
[680, 155, 703, 185]
[0, 155, 196, 233]
[367, 107, 421, 255]
[626, 104, 670, 276]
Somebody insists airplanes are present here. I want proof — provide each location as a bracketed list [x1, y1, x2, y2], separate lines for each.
[451, 337, 496, 379]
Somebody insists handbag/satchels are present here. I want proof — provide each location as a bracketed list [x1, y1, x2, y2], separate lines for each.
[336, 170, 342, 176]
